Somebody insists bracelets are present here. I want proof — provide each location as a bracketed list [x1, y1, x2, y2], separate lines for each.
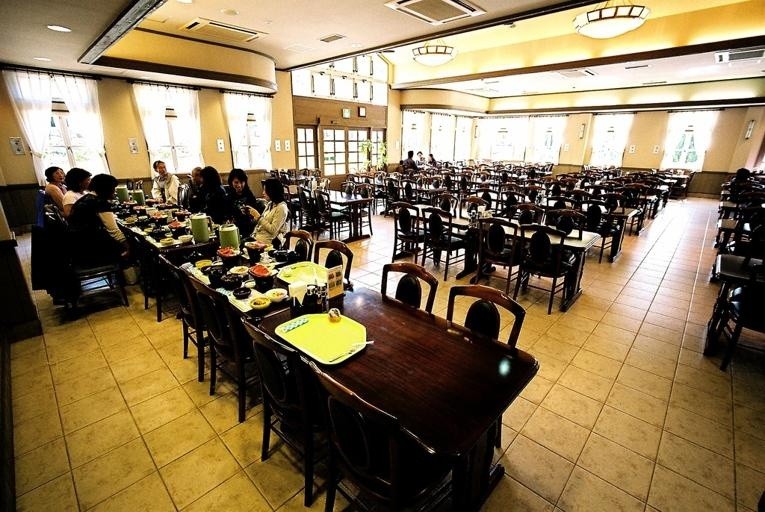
[257, 215, 262, 221]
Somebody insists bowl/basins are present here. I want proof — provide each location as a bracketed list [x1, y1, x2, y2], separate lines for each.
[164, 232, 173, 238]
[245, 241, 268, 258]
[147, 229, 169, 242]
[117, 211, 128, 219]
[195, 259, 212, 269]
[178, 235, 193, 243]
[230, 265, 248, 274]
[133, 208, 148, 217]
[248, 268, 271, 282]
[171, 225, 189, 238]
[160, 237, 174, 246]
[221, 274, 241, 289]
[152, 215, 169, 227]
[250, 297, 270, 310]
[146, 199, 159, 207]
[233, 287, 251, 299]
[265, 287, 287, 302]
[201, 265, 212, 275]
[175, 212, 191, 221]
[217, 248, 244, 265]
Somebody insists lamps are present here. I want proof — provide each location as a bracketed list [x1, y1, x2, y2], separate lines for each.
[413, 34, 457, 67]
[573, 0, 650, 40]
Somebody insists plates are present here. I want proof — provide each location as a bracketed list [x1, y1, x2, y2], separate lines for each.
[228, 289, 262, 313]
[121, 217, 138, 224]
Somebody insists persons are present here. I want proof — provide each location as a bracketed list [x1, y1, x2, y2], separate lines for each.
[223, 168, 256, 239]
[182, 167, 202, 214]
[62, 168, 93, 225]
[150, 160, 180, 207]
[198, 165, 226, 224]
[395, 160, 404, 172]
[62, 174, 143, 286]
[45, 167, 68, 212]
[239, 179, 290, 248]
[404, 150, 423, 174]
[426, 154, 437, 167]
[416, 151, 427, 165]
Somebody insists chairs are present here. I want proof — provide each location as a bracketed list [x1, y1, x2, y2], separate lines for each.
[422, 208, 464, 281]
[390, 201, 424, 264]
[315, 240, 353, 282]
[361, 175, 377, 215]
[240, 315, 332, 506]
[501, 192, 525, 216]
[683, 171, 696, 197]
[512, 224, 576, 315]
[400, 178, 418, 204]
[431, 193, 459, 208]
[346, 174, 359, 183]
[716, 265, 764, 371]
[307, 361, 439, 512]
[158, 255, 211, 382]
[447, 285, 526, 450]
[476, 188, 500, 216]
[188, 275, 260, 423]
[297, 187, 324, 240]
[384, 177, 399, 218]
[283, 230, 314, 259]
[509, 203, 544, 225]
[546, 197, 577, 210]
[381, 264, 438, 314]
[340, 181, 356, 193]
[580, 199, 612, 264]
[282, 185, 302, 231]
[460, 196, 488, 211]
[314, 190, 351, 241]
[565, 190, 594, 200]
[352, 183, 374, 236]
[546, 209, 587, 230]
[597, 193, 629, 207]
[476, 218, 527, 295]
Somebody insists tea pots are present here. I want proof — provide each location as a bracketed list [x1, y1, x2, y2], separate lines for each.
[115, 185, 130, 203]
[216, 223, 240, 250]
[132, 189, 145, 204]
[190, 213, 213, 244]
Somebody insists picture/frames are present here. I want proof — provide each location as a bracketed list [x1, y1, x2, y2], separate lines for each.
[342, 109, 351, 119]
[358, 106, 366, 117]
